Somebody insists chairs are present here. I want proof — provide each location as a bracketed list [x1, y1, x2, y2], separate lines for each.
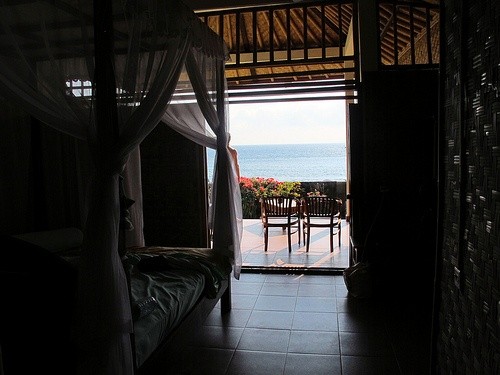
[259, 195, 301, 254]
[303, 197, 341, 253]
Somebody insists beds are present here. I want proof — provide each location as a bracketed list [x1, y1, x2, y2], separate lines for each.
[0, 0, 243, 374]
